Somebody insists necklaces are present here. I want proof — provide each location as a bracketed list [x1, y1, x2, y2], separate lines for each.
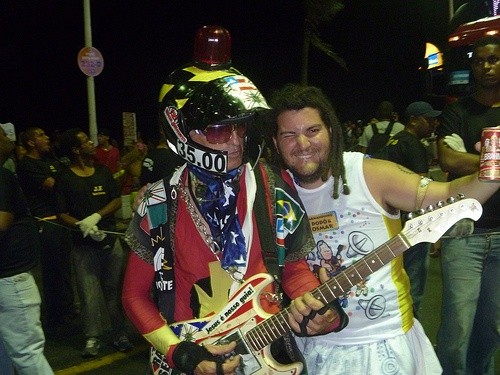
[178, 183, 282, 304]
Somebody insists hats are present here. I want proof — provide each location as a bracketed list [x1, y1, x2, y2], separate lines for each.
[98, 128, 110, 136]
[407, 101, 442, 117]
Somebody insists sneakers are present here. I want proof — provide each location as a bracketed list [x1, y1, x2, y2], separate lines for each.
[113, 333, 134, 351]
[81, 339, 101, 356]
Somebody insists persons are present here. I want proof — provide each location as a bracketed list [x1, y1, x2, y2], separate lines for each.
[0, 62, 494, 375]
[437, 37, 500, 375]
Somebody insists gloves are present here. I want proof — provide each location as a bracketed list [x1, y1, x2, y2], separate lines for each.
[443, 133, 467, 153]
[89, 226, 107, 241]
[75, 213, 102, 238]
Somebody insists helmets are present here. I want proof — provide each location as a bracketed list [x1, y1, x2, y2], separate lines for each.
[158, 66, 256, 174]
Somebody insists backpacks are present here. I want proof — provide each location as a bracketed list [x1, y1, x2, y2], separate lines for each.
[367, 120, 394, 154]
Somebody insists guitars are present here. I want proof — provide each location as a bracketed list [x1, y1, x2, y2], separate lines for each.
[150, 193, 483, 375]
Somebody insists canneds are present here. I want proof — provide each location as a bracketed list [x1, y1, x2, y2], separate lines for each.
[478, 127, 500, 183]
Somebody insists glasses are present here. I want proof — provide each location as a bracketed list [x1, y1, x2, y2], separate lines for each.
[195, 118, 249, 144]
